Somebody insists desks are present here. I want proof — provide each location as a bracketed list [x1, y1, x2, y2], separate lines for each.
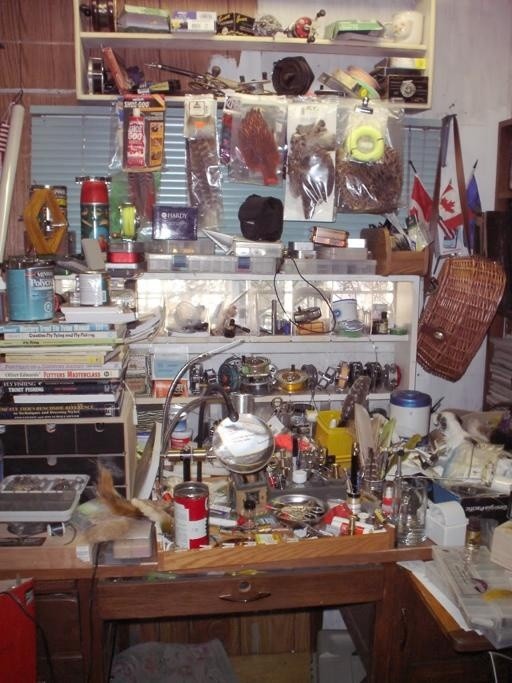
[0, 489, 512, 683]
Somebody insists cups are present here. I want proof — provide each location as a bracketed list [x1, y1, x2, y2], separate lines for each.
[393, 475, 428, 546]
[381, 10, 425, 45]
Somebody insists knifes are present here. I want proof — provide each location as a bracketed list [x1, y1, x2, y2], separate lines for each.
[353, 401, 376, 469]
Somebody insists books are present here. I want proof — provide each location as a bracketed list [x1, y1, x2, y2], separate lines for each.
[1, 320, 132, 417]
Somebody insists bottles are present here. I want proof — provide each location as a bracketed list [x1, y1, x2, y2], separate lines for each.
[239, 500, 257, 532]
[87, 55, 105, 93]
[91, 1, 115, 32]
[80, 176, 109, 262]
[322, 484, 395, 537]
[378, 311, 388, 334]
[464, 516, 481, 551]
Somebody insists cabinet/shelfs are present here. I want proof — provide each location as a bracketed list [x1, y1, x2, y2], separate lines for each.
[372, 564, 499, 683]
[129, 270, 419, 492]
[71, 0, 436, 113]
[32, 580, 95, 683]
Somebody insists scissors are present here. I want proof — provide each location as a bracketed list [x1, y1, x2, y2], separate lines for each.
[272, 500, 324, 521]
[271, 397, 297, 420]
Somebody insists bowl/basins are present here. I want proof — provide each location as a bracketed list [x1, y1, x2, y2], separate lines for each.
[213, 412, 274, 475]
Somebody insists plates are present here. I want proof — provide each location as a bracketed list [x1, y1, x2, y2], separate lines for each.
[264, 492, 332, 526]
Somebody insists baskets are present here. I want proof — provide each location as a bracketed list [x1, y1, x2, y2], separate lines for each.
[417, 255, 507, 382]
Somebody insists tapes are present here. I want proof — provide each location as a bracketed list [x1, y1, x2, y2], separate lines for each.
[430, 500, 466, 526]
